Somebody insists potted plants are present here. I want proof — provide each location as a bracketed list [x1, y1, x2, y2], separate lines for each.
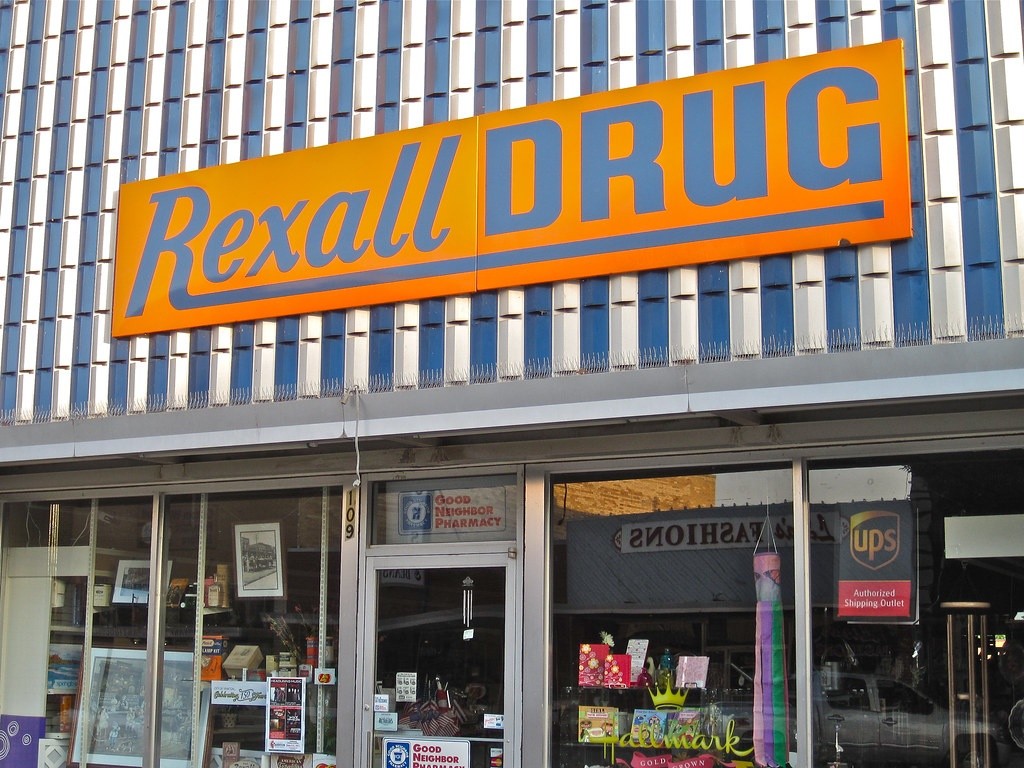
[220, 705, 238, 728]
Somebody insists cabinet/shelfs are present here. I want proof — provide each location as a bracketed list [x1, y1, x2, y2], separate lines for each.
[559, 686, 753, 768]
[0, 547, 283, 768]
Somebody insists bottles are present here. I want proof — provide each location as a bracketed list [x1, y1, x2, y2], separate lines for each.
[436, 682, 448, 712]
[637, 666, 653, 688]
[660, 648, 676, 669]
[205, 574, 224, 607]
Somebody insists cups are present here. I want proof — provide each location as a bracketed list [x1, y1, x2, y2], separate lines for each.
[657, 669, 672, 689]
[220, 713, 237, 728]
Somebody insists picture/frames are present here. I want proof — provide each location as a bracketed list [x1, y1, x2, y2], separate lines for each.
[113, 560, 173, 604]
[68, 641, 218, 768]
[47, 639, 84, 689]
[230, 518, 288, 600]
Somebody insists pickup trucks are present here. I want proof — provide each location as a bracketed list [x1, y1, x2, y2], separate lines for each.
[706, 670, 1006, 768]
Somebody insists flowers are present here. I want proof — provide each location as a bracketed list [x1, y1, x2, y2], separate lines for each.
[261, 611, 307, 663]
[294, 605, 318, 638]
[600, 630, 615, 655]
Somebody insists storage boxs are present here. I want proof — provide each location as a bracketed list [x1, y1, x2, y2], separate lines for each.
[222, 645, 264, 678]
[266, 655, 279, 677]
[631, 709, 668, 743]
[270, 752, 336, 768]
[201, 636, 230, 681]
[676, 656, 709, 688]
[579, 643, 632, 689]
[60, 696, 72, 731]
[223, 742, 241, 768]
[94, 584, 113, 606]
